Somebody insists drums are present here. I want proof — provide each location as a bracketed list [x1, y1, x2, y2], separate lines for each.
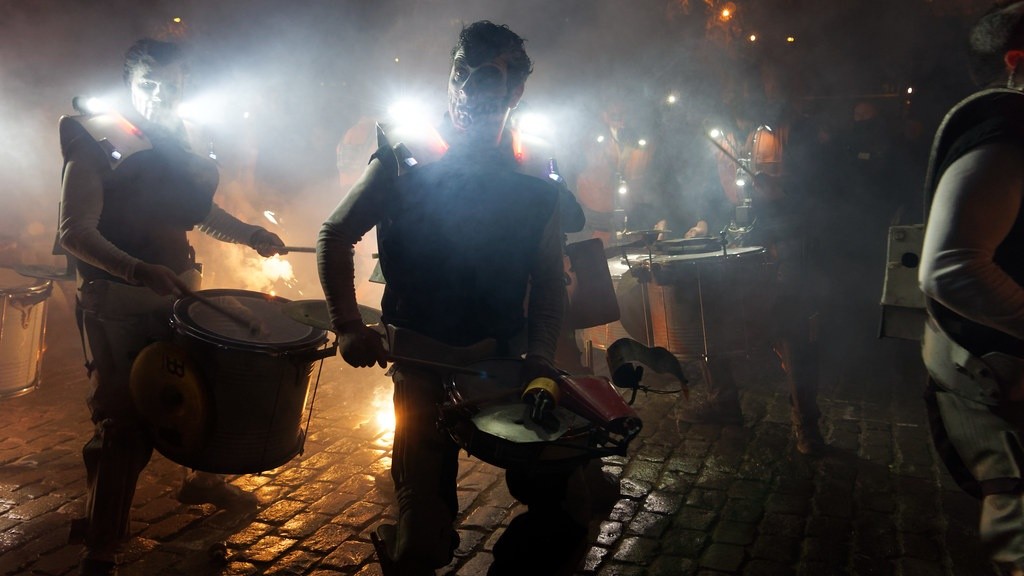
[157, 288, 328, 476]
[1, 265, 53, 401]
[639, 245, 777, 360]
[442, 355, 600, 468]
[582, 248, 664, 350]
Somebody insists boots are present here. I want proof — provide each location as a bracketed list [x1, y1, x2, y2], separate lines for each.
[783, 340, 826, 455]
[678, 357, 743, 423]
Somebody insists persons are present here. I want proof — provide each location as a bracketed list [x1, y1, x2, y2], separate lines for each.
[50, 39, 287, 576]
[318, 23, 897, 575]
[916, 33, 1024, 574]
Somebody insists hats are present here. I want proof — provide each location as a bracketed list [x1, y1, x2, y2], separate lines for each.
[1005, 17, 1024, 68]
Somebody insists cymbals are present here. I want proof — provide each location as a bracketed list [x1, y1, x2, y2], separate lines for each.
[17, 263, 76, 281]
[282, 298, 380, 332]
[625, 228, 672, 237]
[662, 236, 720, 244]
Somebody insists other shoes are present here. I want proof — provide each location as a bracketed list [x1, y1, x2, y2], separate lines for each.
[176, 474, 258, 511]
[79, 559, 117, 576]
